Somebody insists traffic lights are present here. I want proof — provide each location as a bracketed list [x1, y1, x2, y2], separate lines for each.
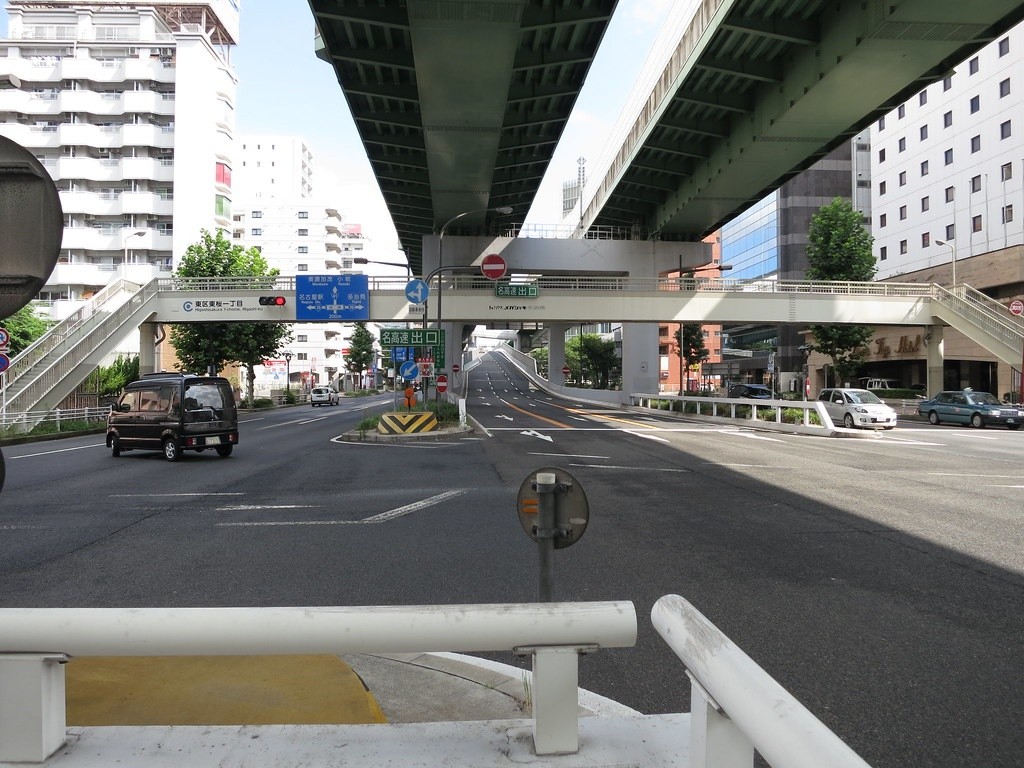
[258, 296, 286, 305]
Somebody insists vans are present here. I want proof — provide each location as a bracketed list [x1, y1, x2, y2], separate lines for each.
[104, 371, 240, 461]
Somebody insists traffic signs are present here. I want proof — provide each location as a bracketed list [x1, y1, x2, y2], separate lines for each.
[296, 275, 370, 321]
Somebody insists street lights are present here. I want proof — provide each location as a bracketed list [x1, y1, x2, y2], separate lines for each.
[354, 247, 411, 409]
[935, 239, 956, 296]
[282, 350, 295, 395]
[679, 254, 733, 411]
[433, 205, 514, 419]
[124, 230, 147, 283]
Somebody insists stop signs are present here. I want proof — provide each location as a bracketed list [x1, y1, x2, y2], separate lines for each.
[453, 364, 459, 372]
[481, 254, 507, 280]
[562, 367, 570, 374]
[806, 377, 810, 396]
[436, 375, 448, 394]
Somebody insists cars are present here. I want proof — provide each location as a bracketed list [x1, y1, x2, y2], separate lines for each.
[859, 376, 928, 391]
[309, 386, 339, 408]
[815, 387, 897, 429]
[727, 383, 786, 411]
[917, 387, 1024, 430]
